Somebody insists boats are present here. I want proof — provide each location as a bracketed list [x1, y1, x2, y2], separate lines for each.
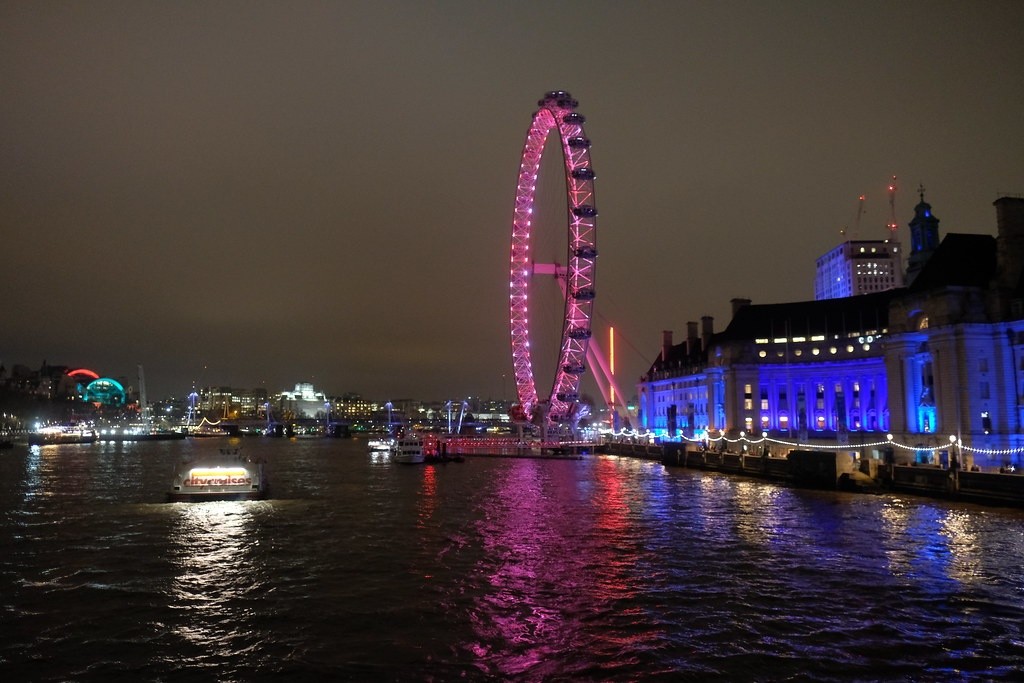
[27, 422, 101, 445]
[169, 438, 266, 503]
[362, 401, 394, 456]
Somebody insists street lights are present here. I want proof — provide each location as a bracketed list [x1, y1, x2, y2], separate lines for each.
[679, 429, 685, 447]
[644, 428, 651, 444]
[719, 430, 729, 453]
[703, 426, 712, 449]
[620, 427, 625, 443]
[737, 427, 747, 451]
[759, 431, 769, 460]
[881, 433, 896, 465]
[946, 433, 959, 475]
[662, 430, 667, 447]
[632, 428, 639, 446]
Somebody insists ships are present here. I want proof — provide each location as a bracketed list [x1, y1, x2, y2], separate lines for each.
[394, 430, 426, 465]
